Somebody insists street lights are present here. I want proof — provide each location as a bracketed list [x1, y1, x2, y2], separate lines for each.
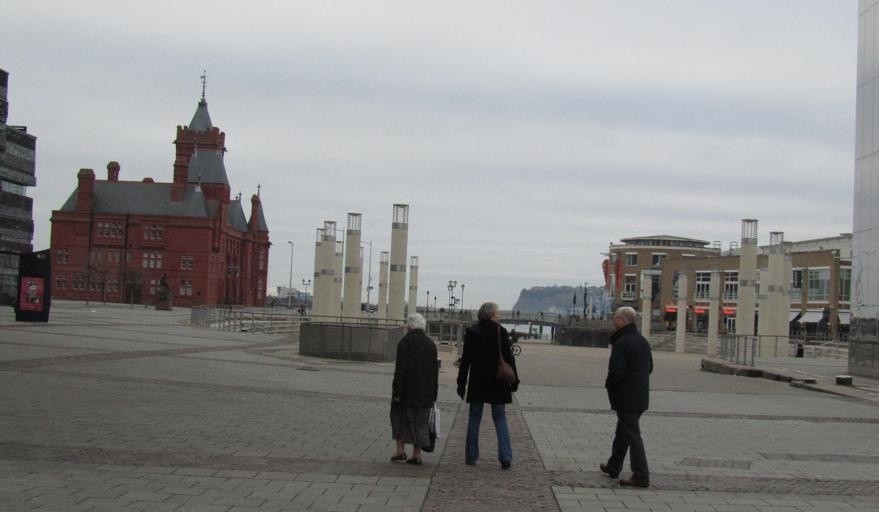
[434, 296, 437, 309]
[426, 290, 430, 319]
[288, 241, 293, 308]
[302, 279, 312, 304]
[447, 280, 466, 314]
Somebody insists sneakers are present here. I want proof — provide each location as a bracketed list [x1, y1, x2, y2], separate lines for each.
[502, 459, 511, 469]
[600, 463, 619, 479]
[619, 477, 648, 487]
[391, 452, 421, 465]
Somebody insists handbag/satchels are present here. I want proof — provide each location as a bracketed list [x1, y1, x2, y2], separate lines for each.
[497, 355, 517, 384]
[423, 401, 442, 453]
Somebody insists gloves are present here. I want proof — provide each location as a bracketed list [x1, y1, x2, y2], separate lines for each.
[457, 385, 465, 400]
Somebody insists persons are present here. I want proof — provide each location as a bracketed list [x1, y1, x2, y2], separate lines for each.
[390, 313, 439, 465]
[516, 309, 521, 319]
[511, 310, 515, 320]
[599, 305, 654, 489]
[540, 311, 544, 319]
[558, 313, 562, 322]
[795, 343, 804, 358]
[456, 303, 521, 469]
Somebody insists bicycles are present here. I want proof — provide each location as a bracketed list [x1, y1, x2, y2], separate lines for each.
[509, 341, 522, 356]
